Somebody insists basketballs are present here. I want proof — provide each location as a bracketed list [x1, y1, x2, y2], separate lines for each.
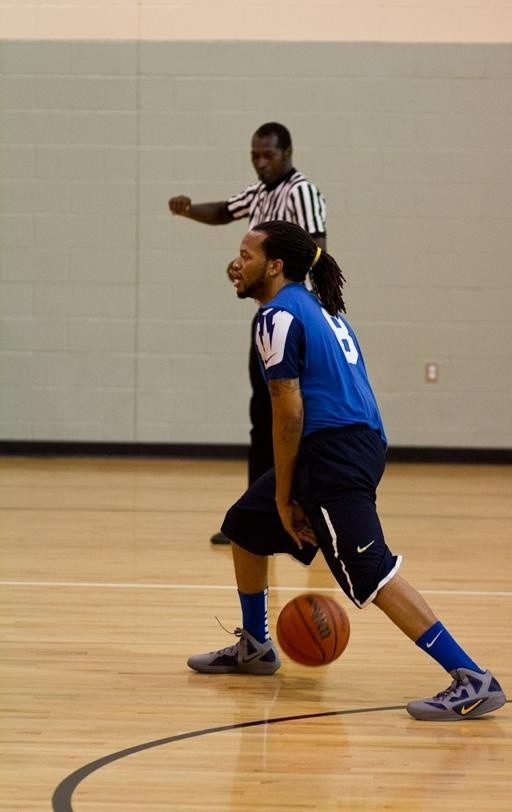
[277, 595, 348, 664]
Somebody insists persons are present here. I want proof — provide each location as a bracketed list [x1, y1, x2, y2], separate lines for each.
[183, 220, 508, 721]
[167, 120, 328, 547]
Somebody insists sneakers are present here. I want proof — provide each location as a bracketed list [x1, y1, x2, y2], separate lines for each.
[186, 629, 281, 676]
[407, 667, 507, 721]
